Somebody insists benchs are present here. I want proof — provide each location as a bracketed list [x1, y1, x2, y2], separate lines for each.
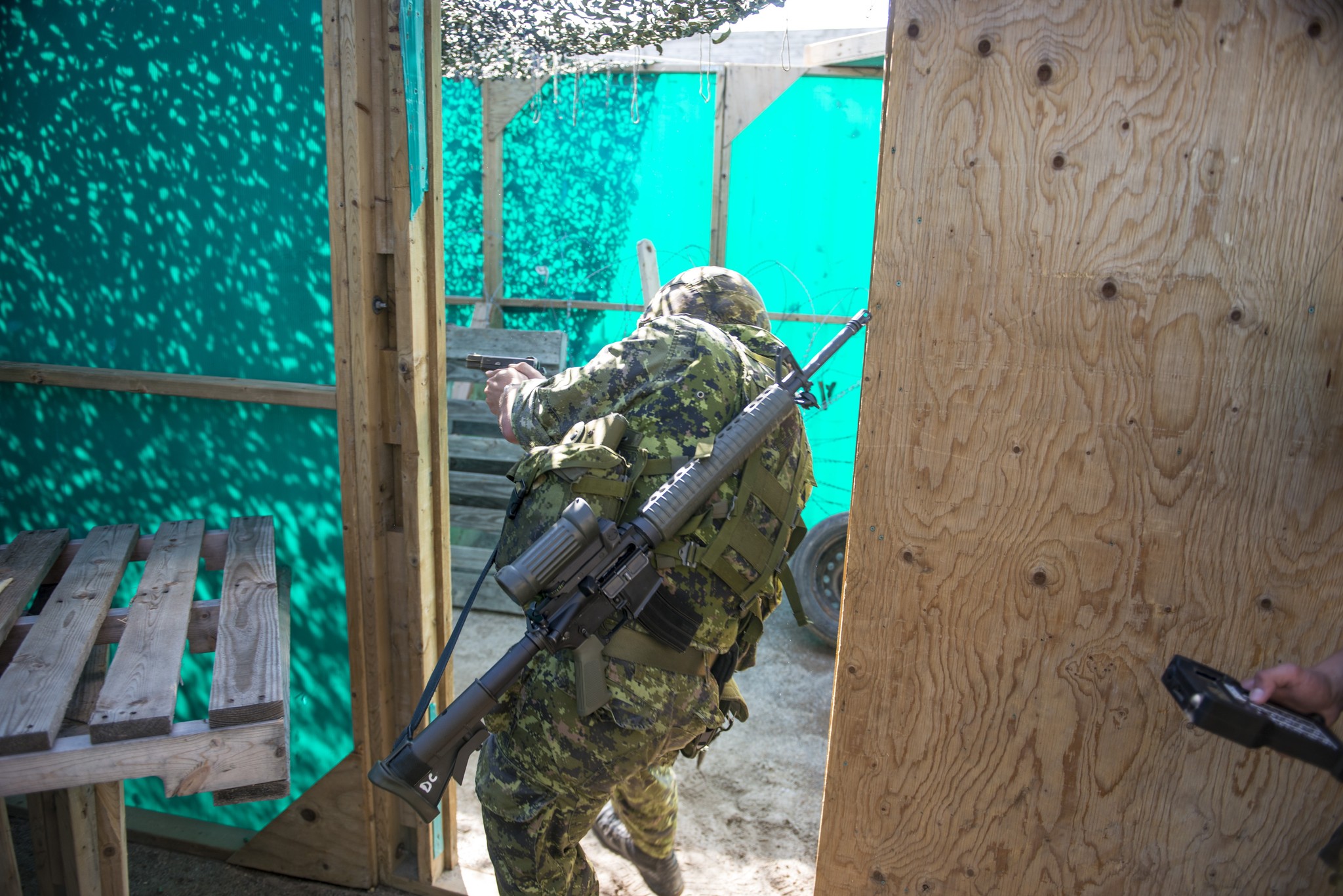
[1, 516, 295, 896]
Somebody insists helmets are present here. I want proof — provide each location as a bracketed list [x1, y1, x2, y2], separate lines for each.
[637, 264, 771, 335]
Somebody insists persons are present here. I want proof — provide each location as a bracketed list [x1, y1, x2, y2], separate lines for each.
[474, 261, 817, 894]
[1240, 644, 1343, 887]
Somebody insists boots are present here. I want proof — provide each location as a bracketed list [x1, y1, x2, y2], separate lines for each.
[593, 800, 684, 896]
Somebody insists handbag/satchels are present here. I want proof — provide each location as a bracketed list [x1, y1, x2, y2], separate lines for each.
[495, 443, 648, 587]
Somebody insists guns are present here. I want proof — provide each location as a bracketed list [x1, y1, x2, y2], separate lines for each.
[371, 308, 870, 824]
[464, 352, 540, 374]
